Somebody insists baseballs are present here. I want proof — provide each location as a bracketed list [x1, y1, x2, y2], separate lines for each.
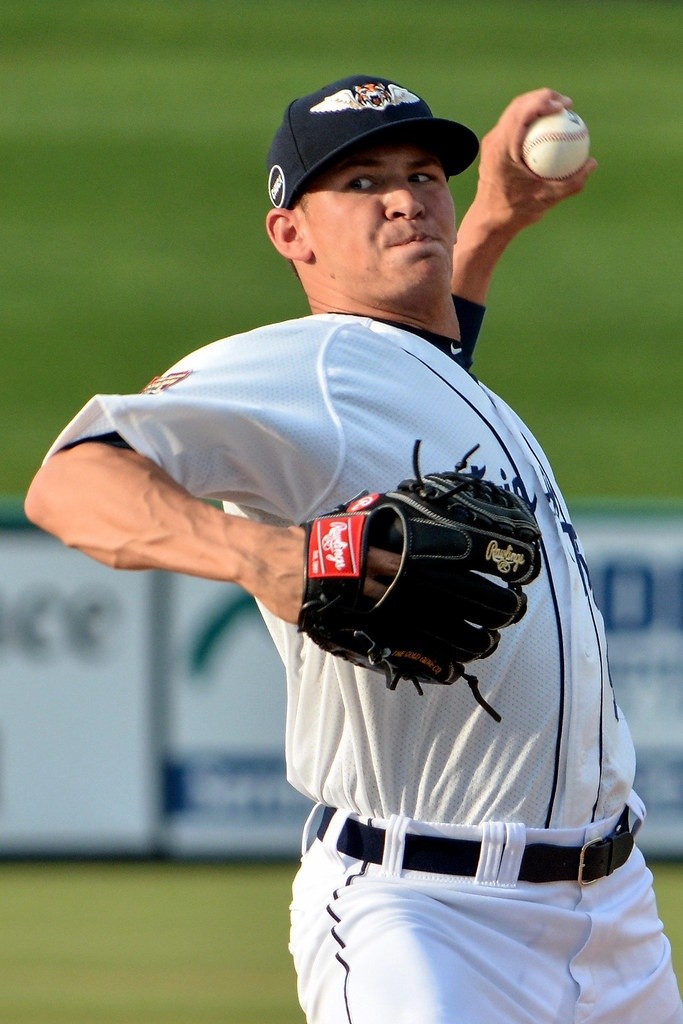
[523, 105, 588, 179]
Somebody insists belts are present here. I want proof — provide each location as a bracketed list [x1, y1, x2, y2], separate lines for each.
[308, 802, 646, 882]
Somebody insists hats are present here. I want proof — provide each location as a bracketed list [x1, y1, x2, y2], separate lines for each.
[264, 76, 481, 211]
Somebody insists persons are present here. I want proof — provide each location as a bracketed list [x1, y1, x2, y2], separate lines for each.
[25, 76, 681, 1024]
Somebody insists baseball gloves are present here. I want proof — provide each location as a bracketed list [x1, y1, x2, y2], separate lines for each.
[296, 433, 544, 725]
